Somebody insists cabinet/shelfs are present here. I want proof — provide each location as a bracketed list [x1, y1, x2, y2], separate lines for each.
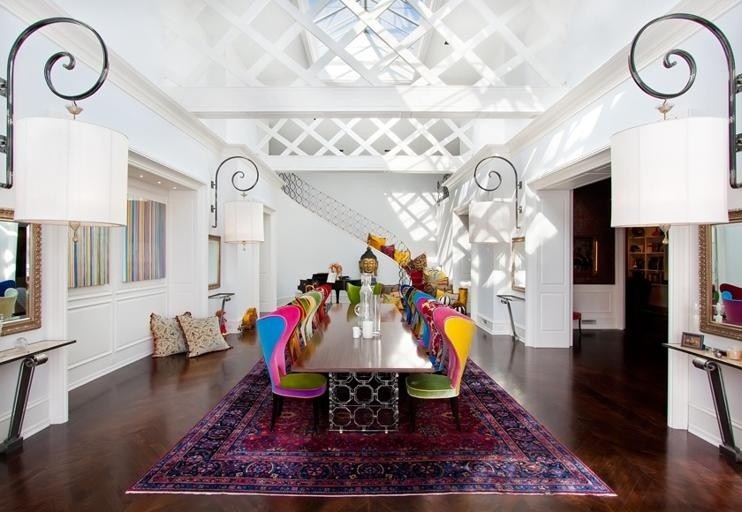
[625, 227, 668, 308]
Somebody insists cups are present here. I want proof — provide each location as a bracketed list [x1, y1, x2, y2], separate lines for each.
[362, 320, 373, 339]
[352, 326, 361, 339]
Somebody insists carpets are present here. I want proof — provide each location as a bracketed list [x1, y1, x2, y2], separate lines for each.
[124, 355, 618, 497]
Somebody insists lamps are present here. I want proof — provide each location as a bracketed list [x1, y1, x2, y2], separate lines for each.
[609, 13, 742, 245]
[468, 156, 522, 246]
[0, 17, 129, 243]
[592, 236, 602, 276]
[211, 156, 264, 251]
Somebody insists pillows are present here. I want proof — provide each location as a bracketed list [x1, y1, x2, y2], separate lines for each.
[149, 311, 231, 359]
[366, 233, 427, 273]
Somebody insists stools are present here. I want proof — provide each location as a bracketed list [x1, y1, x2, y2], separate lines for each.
[573, 312, 582, 333]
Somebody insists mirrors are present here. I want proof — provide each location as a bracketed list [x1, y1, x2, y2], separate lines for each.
[0, 210, 42, 337]
[697, 209, 742, 341]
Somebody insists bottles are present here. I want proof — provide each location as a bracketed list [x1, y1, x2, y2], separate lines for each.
[354, 282, 381, 340]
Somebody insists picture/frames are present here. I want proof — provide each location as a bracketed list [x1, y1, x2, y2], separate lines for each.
[511, 237, 525, 294]
[681, 332, 704, 349]
[208, 235, 221, 291]
[573, 228, 599, 282]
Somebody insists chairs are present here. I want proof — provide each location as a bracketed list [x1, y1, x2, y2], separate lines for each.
[410, 272, 434, 293]
[0, 288, 19, 316]
[255, 283, 475, 433]
[719, 281, 742, 326]
